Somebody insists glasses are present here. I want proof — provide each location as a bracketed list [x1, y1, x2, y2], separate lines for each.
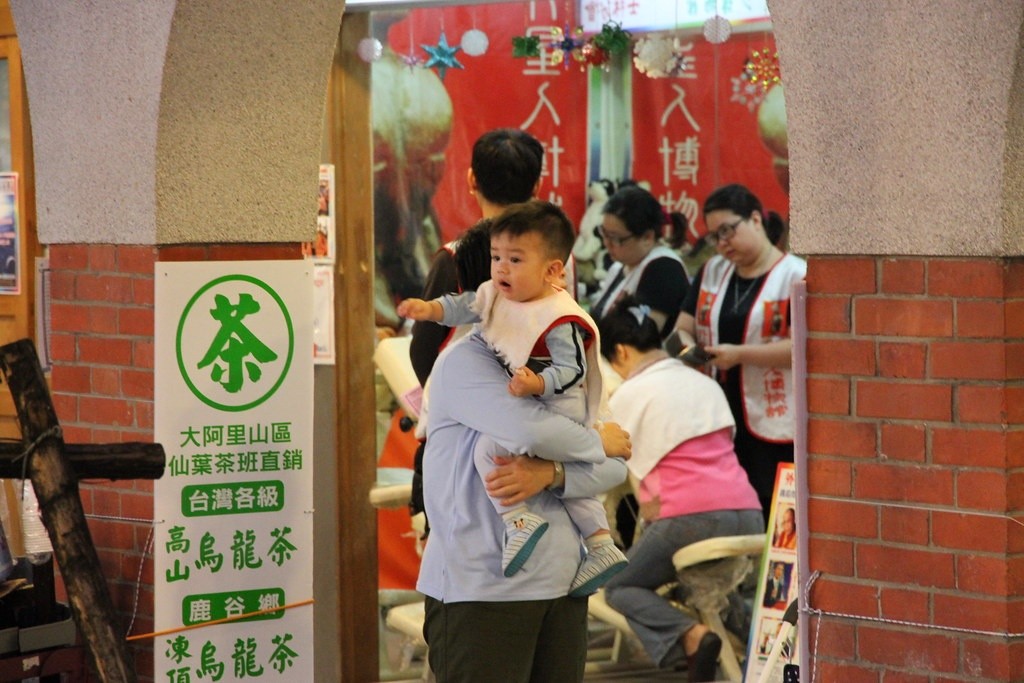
[704, 217, 745, 247]
[597, 225, 635, 250]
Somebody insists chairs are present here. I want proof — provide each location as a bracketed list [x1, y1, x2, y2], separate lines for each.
[369, 484, 433, 681]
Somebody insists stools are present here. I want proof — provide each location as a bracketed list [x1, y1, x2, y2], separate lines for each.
[585, 533, 766, 683]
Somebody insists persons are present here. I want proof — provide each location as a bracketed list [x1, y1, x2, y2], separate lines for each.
[408, 128, 578, 543]
[764, 563, 788, 606]
[773, 508, 796, 549]
[590, 185, 692, 341]
[595, 299, 765, 683]
[758, 622, 790, 656]
[414, 215, 634, 683]
[396, 201, 629, 598]
[679, 183, 808, 534]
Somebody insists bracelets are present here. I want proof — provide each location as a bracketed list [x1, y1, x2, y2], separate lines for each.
[548, 461, 564, 491]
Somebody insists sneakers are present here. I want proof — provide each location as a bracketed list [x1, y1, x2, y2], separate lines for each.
[568, 542, 629, 599]
[501, 512, 549, 578]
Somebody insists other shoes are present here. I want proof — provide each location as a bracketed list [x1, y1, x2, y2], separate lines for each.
[687, 632, 722, 683]
